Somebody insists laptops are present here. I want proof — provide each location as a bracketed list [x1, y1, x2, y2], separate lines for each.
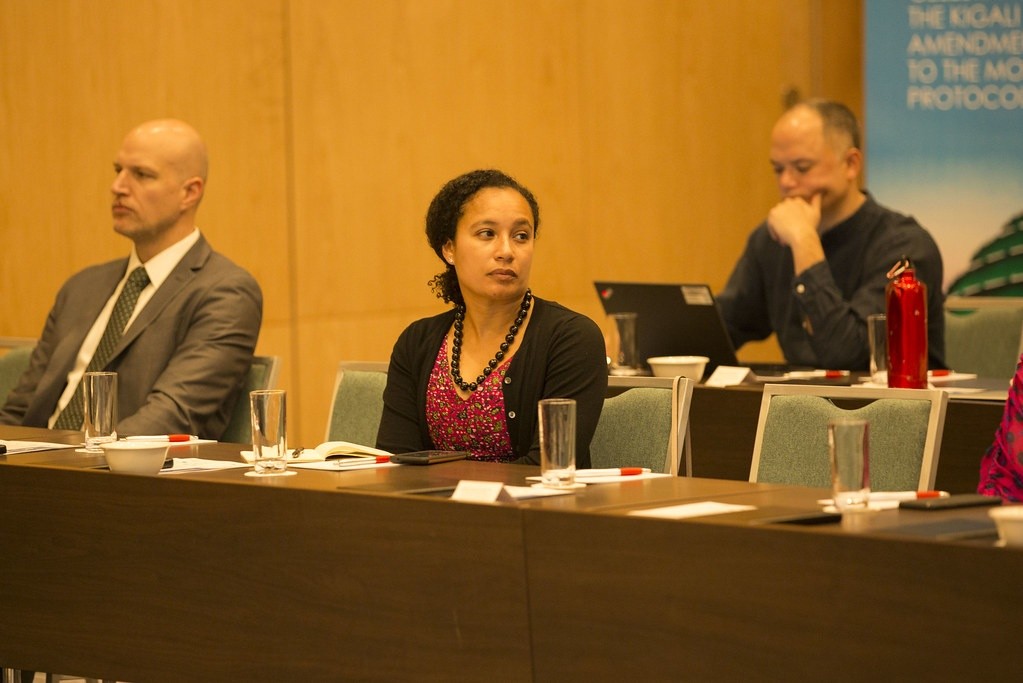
[592, 280, 815, 378]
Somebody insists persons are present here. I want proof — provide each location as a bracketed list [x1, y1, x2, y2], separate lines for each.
[0, 118, 263, 442]
[374, 169, 609, 468]
[710, 98, 947, 369]
[977, 353, 1023, 507]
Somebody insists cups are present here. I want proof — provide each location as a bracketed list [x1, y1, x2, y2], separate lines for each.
[867, 315, 888, 385]
[538, 398, 576, 485]
[82, 372, 118, 452]
[607, 312, 637, 376]
[828, 418, 871, 510]
[250, 390, 287, 473]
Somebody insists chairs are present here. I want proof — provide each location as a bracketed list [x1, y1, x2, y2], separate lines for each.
[0, 337, 38, 408]
[324, 361, 391, 449]
[942, 294, 1023, 379]
[588, 376, 693, 476]
[749, 384, 947, 491]
[220, 354, 277, 446]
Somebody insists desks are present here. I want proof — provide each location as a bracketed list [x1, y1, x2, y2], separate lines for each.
[0, 375, 1023, 683]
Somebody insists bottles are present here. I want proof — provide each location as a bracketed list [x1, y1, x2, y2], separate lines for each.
[885, 255, 928, 390]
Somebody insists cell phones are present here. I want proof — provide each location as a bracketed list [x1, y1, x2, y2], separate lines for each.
[899, 493, 1002, 510]
[389, 450, 467, 464]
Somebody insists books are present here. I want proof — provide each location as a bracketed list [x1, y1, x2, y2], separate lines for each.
[240, 441, 395, 464]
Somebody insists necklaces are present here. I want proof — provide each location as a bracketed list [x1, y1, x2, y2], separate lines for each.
[451, 288, 532, 390]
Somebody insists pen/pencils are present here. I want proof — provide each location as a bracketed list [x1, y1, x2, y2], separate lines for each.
[292, 446, 305, 457]
[340, 455, 390, 466]
[840, 490, 951, 502]
[126, 433, 189, 442]
[815, 369, 850, 376]
[576, 467, 651, 477]
[927, 369, 954, 376]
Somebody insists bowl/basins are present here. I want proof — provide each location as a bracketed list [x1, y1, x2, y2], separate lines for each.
[987, 506, 1023, 548]
[647, 355, 709, 383]
[100, 442, 171, 475]
